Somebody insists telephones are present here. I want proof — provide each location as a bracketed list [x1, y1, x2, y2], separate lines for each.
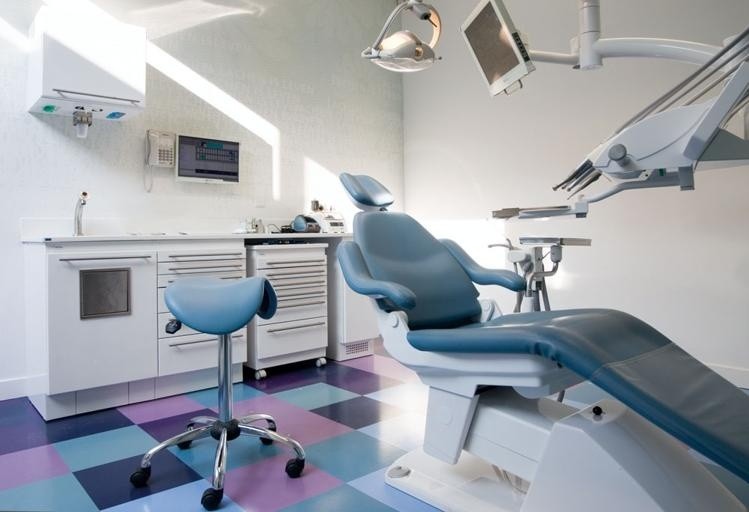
[145, 129, 176, 168]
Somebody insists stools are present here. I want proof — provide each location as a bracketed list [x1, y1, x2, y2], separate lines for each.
[126, 274, 307, 511]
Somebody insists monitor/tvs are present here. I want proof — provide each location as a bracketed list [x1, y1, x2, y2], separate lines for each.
[460, 0, 535, 96]
[174, 133, 240, 184]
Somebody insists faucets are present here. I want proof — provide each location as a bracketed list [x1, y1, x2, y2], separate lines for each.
[72, 191, 87, 236]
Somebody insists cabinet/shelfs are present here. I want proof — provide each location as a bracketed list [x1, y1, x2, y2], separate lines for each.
[156, 240, 246, 401]
[246, 242, 330, 382]
[18, 242, 155, 423]
[294, 237, 380, 362]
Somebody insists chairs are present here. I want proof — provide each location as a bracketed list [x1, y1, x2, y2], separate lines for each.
[332, 165, 749, 512]
